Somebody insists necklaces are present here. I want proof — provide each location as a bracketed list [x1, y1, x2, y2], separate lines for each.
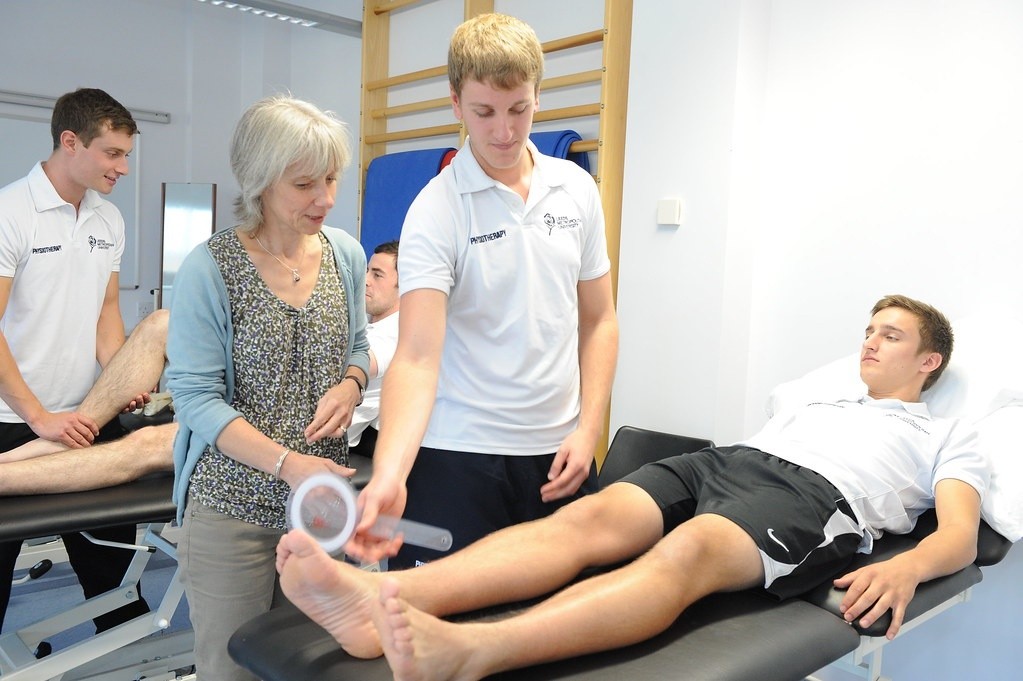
[251, 228, 310, 283]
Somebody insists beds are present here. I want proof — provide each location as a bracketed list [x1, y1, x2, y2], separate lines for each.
[228, 420, 1014, 681]
[0, 450, 375, 681]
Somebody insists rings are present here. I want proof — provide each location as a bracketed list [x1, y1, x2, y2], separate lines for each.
[340, 426, 347, 433]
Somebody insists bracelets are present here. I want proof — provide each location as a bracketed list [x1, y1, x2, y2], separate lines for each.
[276, 446, 291, 482]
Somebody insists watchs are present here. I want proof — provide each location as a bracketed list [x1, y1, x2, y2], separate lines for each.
[338, 375, 365, 407]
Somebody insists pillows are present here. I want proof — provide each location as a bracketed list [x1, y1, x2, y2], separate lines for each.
[775, 340, 1023, 542]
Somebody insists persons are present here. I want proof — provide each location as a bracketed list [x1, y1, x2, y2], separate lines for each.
[0, 89, 151, 639]
[341, 11, 618, 622]
[276, 292, 992, 680]
[174, 96, 375, 681]
[0, 240, 401, 496]
